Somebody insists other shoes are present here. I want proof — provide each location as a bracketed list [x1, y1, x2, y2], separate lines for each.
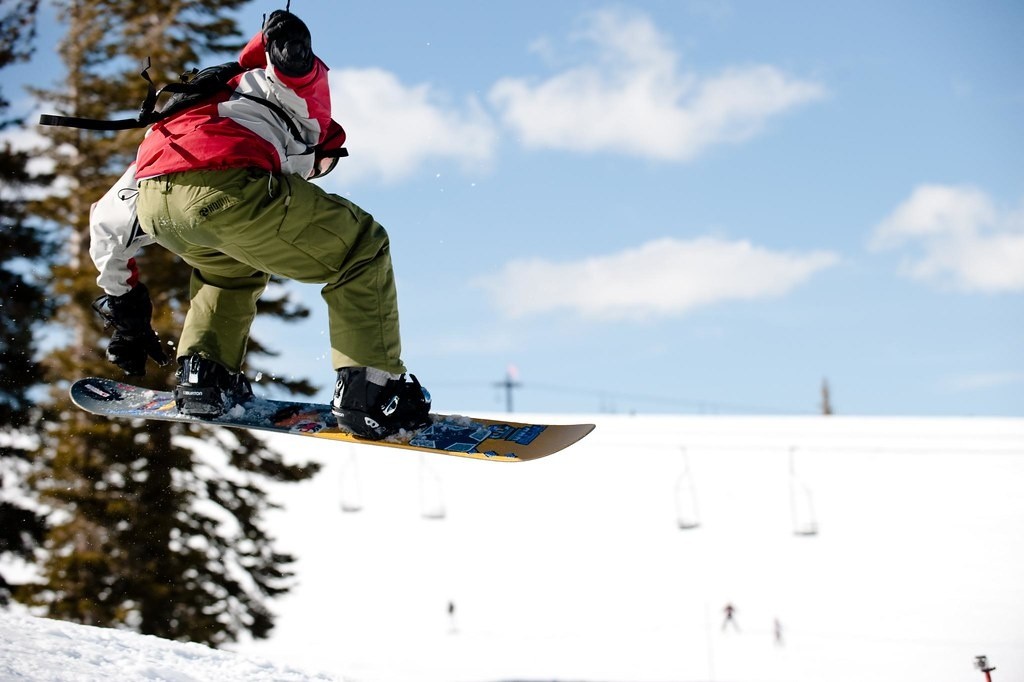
[175, 352, 251, 417]
[330, 362, 431, 440]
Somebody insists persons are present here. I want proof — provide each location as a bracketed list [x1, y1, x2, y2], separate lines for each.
[88, 9, 436, 439]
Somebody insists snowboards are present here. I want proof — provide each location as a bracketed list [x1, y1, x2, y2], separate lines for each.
[68, 377, 597, 464]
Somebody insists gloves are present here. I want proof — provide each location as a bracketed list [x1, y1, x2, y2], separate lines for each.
[93, 282, 168, 378]
[262, 9, 314, 77]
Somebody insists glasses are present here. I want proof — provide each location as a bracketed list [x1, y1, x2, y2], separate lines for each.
[307, 156, 339, 180]
